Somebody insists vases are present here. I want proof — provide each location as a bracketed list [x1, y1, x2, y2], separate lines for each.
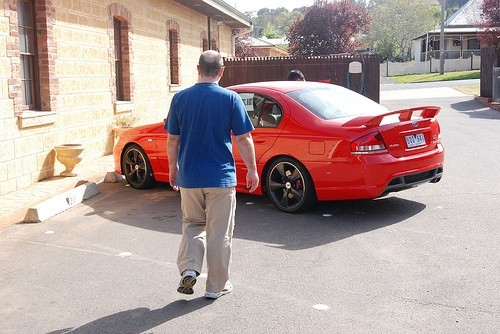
[54, 143, 84, 176]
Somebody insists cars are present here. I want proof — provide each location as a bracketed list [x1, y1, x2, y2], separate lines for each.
[114, 82, 445, 214]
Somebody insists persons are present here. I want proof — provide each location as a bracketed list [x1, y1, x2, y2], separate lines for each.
[163, 49, 259, 299]
[288, 69, 307, 82]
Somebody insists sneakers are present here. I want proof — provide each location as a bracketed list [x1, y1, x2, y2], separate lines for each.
[204, 280, 233, 299]
[176, 269, 197, 295]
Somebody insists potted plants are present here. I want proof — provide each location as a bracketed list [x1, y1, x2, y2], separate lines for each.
[113, 116, 141, 139]
